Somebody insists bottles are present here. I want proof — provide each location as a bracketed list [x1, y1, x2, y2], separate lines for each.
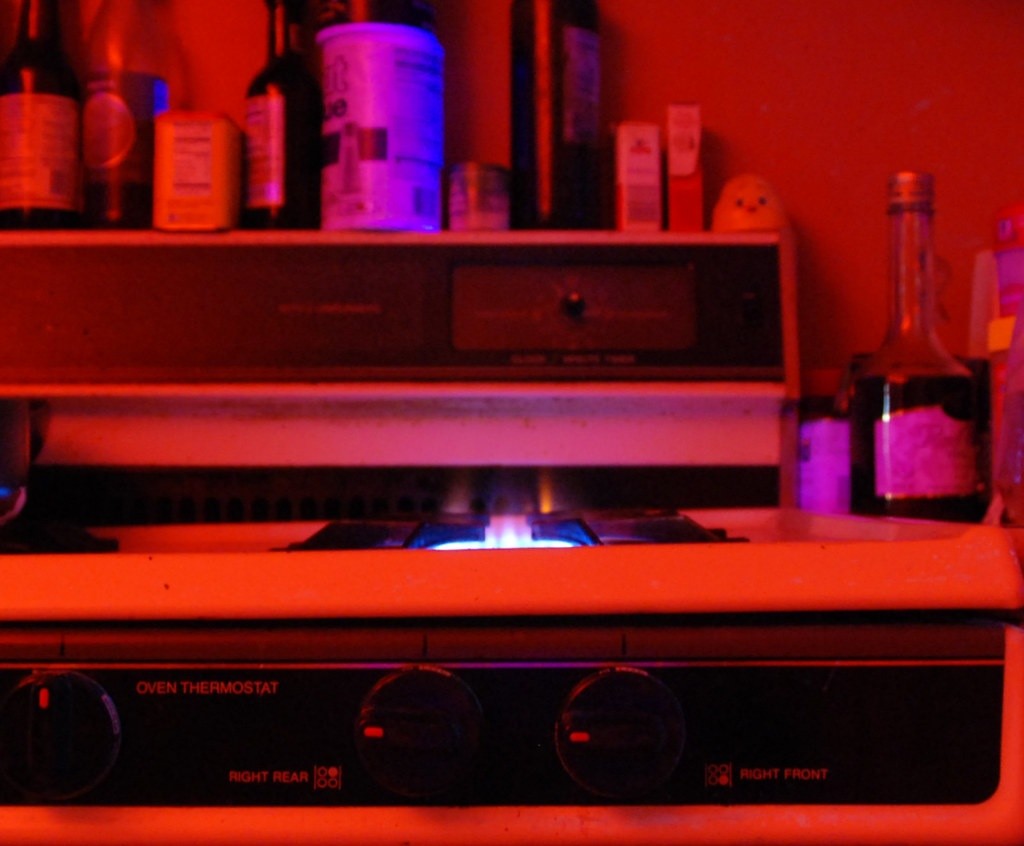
[238, 3, 319, 226]
[3, 0, 178, 232]
[850, 168, 986, 517]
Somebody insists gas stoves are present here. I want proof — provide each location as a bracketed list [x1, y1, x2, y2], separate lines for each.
[0, 232, 1020, 846]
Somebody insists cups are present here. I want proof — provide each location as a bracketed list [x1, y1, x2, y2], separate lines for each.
[316, 23, 445, 231]
[794, 364, 850, 516]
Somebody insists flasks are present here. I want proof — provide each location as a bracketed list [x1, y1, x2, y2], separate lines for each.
[506, 2, 603, 229]
